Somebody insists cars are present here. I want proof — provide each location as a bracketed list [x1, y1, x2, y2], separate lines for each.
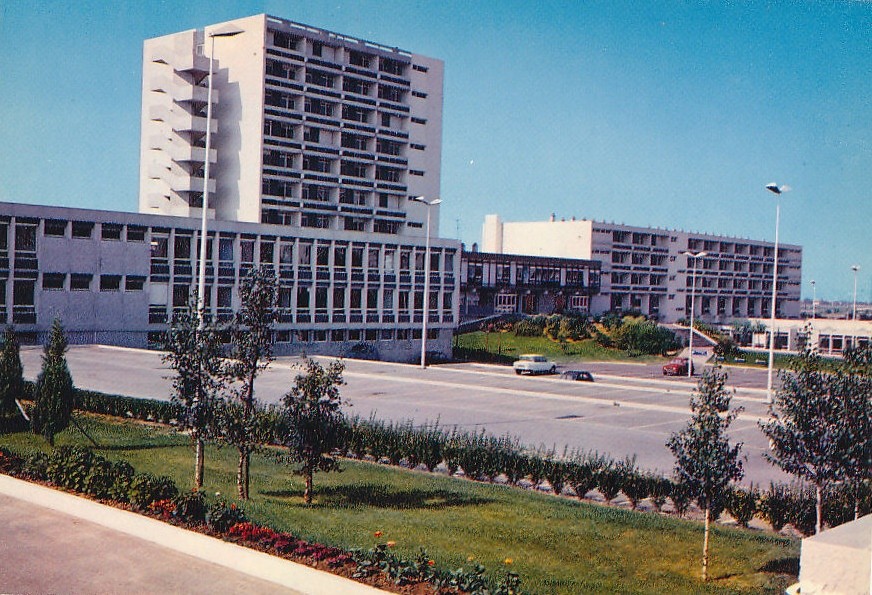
[513, 354, 557, 375]
[558, 371, 594, 383]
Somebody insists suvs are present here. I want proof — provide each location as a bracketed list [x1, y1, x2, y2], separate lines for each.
[663, 358, 694, 376]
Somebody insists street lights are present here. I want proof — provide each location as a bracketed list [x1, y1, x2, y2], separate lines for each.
[764, 183, 790, 402]
[810, 280, 816, 328]
[415, 197, 443, 371]
[193, 23, 246, 409]
[683, 251, 709, 377]
[851, 266, 860, 351]
